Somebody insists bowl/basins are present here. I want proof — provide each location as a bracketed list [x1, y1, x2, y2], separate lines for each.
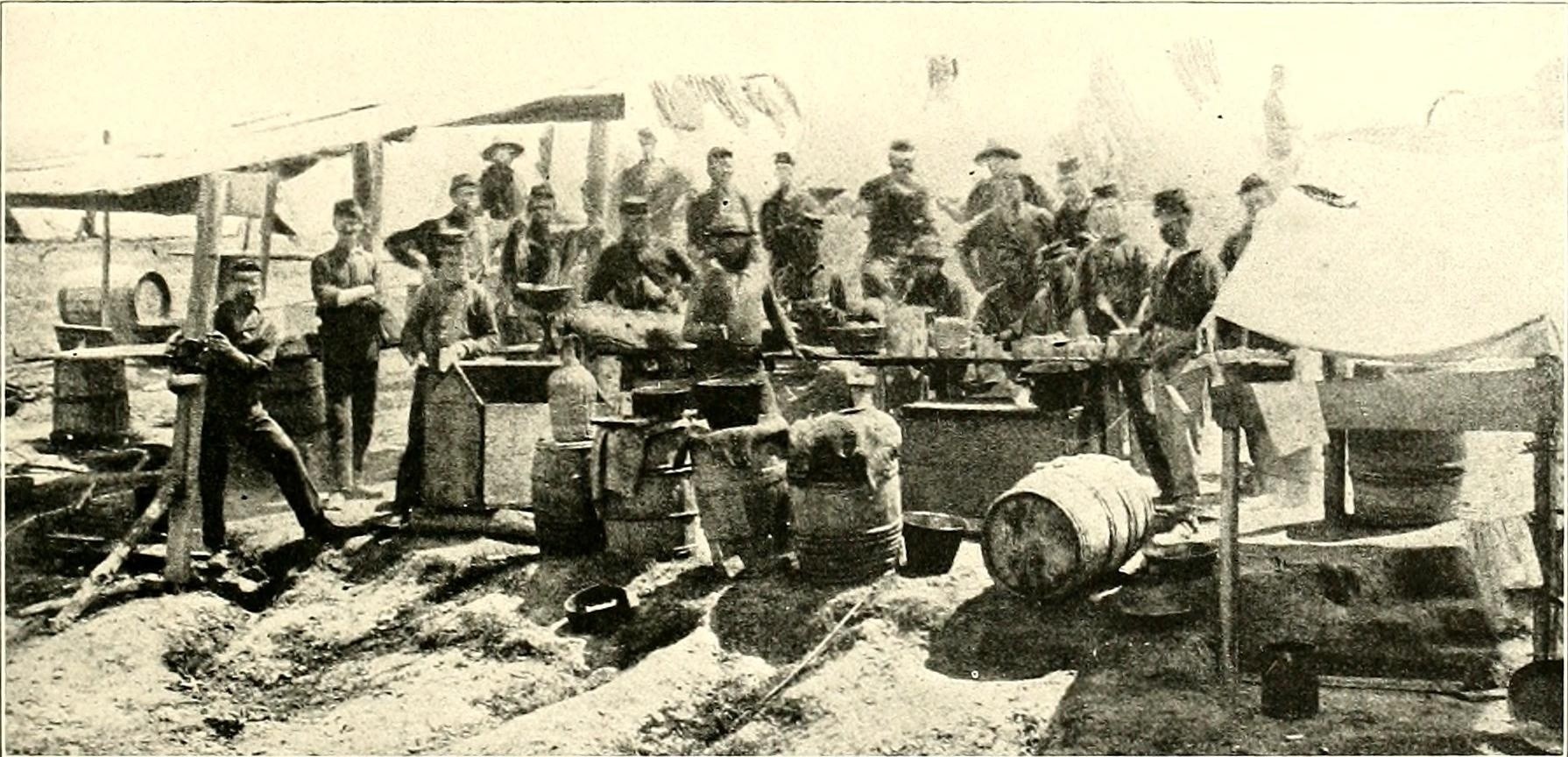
[565, 582, 632, 632]
[1117, 591, 1199, 623]
[828, 325, 885, 355]
[630, 385, 685, 422]
[1508, 659, 1563, 754]
[1142, 541, 1215, 576]
[696, 380, 760, 427]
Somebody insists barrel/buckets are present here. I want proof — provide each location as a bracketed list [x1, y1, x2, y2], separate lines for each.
[983, 453, 1154, 603]
[52, 357, 130, 435]
[532, 438, 608, 552]
[1347, 360, 1469, 529]
[1260, 642, 1320, 721]
[597, 426, 701, 567]
[259, 361, 322, 475]
[688, 425, 799, 584]
[787, 409, 904, 585]
[893, 512, 970, 577]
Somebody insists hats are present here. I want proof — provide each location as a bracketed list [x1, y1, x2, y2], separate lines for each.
[1094, 185, 1119, 199]
[1152, 189, 1188, 224]
[482, 137, 525, 162]
[1237, 173, 1264, 196]
[1056, 156, 1080, 175]
[335, 200, 364, 219]
[975, 142, 1023, 164]
[774, 152, 792, 164]
[906, 238, 948, 263]
[701, 212, 755, 235]
[619, 199, 648, 215]
[450, 174, 475, 195]
[1033, 240, 1074, 269]
[231, 258, 260, 274]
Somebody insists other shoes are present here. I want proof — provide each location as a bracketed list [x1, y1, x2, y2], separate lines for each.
[1152, 491, 1203, 516]
[325, 490, 346, 511]
[208, 553, 230, 570]
[312, 524, 353, 545]
[348, 483, 383, 499]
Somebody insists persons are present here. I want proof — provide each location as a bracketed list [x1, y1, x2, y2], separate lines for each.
[683, 144, 820, 360]
[494, 179, 589, 319]
[608, 126, 700, 238]
[589, 194, 703, 313]
[383, 175, 508, 298]
[158, 260, 344, 546]
[396, 227, 508, 518]
[856, 137, 1307, 534]
[309, 199, 397, 499]
[475, 131, 531, 216]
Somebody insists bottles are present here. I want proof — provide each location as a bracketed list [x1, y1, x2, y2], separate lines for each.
[547, 343, 593, 444]
